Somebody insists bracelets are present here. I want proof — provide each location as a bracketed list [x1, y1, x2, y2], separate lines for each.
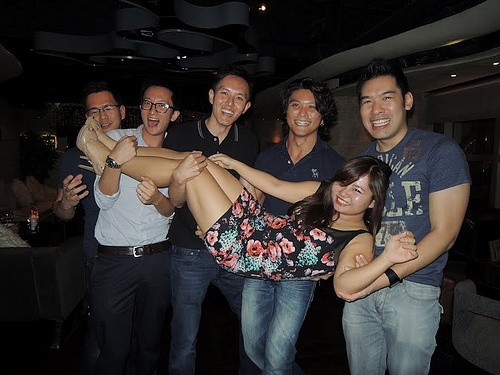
[60, 202, 73, 211]
[157, 194, 163, 207]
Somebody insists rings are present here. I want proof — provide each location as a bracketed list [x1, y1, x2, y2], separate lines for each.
[139, 191, 143, 196]
[63, 194, 70, 201]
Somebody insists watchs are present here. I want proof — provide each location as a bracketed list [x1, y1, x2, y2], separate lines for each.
[106, 155, 122, 169]
[385, 268, 402, 288]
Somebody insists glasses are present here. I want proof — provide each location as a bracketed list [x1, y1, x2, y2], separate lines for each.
[86, 105, 120, 117]
[139, 100, 175, 114]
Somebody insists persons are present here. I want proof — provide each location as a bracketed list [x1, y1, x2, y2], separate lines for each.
[195, 77, 350, 375]
[76, 116, 418, 299]
[342, 57, 473, 375]
[93, 81, 180, 375]
[51, 82, 126, 279]
[162, 66, 260, 375]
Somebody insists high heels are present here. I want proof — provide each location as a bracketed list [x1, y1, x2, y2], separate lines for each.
[79, 116, 102, 160]
[76, 123, 104, 175]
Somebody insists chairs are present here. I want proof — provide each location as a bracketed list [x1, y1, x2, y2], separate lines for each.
[439, 199, 500, 375]
[0, 235, 91, 352]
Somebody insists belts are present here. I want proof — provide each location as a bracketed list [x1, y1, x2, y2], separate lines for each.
[98, 240, 172, 258]
